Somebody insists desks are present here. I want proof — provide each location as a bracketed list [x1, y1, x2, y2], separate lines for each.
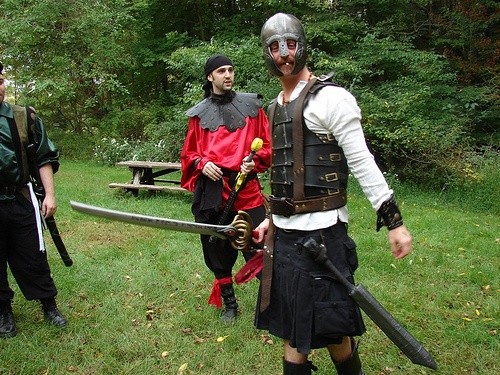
[118, 161, 180, 197]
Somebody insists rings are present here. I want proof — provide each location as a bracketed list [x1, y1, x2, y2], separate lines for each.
[206, 173, 209, 175]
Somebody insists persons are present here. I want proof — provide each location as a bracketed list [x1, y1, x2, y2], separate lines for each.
[0, 62, 67, 338]
[252, 13, 412, 375]
[181, 55, 272, 322]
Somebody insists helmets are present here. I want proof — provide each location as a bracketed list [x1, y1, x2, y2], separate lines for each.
[262, 13, 306, 77]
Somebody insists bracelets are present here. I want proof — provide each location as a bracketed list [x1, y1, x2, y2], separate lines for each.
[376, 196, 403, 232]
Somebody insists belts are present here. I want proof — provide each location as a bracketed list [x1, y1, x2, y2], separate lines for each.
[260, 193, 348, 312]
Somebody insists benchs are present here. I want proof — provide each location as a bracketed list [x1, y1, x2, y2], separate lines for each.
[109, 183, 188, 192]
[131, 177, 181, 184]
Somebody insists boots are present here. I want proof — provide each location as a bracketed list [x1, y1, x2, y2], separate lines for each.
[0, 300, 18, 337]
[331, 337, 364, 375]
[282, 356, 317, 375]
[40, 295, 68, 327]
[217, 274, 237, 321]
